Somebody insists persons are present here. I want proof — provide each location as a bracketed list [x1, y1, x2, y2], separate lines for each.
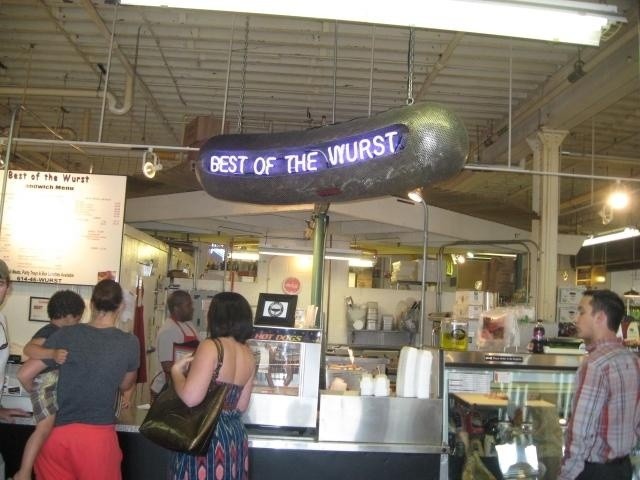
[148, 289, 201, 402]
[554, 289, 640, 480]
[164, 290, 257, 480]
[1, 258, 34, 480]
[13, 289, 85, 480]
[15, 278, 143, 479]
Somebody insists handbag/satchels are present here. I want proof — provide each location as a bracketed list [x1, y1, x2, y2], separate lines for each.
[138, 372, 229, 457]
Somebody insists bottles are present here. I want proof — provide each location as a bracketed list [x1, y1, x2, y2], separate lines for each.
[533, 318, 546, 354]
